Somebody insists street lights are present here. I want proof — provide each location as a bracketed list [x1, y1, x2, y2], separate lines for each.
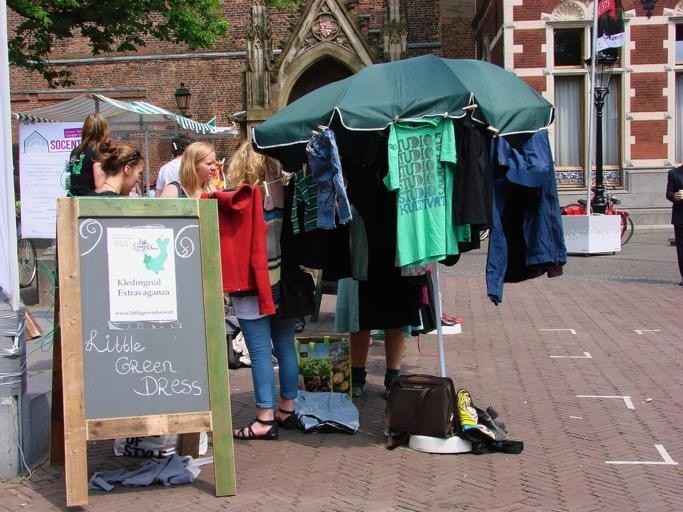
[581, 49, 620, 213]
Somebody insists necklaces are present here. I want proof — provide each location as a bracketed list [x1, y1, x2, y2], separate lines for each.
[102, 182, 117, 194]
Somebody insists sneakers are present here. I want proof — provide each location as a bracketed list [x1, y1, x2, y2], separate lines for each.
[295, 317, 305, 332]
[352, 386, 363, 397]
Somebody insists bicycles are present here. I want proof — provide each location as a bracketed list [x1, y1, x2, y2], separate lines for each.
[14, 231, 37, 289]
[559, 197, 634, 247]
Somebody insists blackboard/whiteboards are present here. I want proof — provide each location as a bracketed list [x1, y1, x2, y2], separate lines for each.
[57, 196, 234, 437]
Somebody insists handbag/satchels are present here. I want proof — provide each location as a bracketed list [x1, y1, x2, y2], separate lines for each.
[295, 336, 352, 399]
[388, 374, 457, 437]
[279, 271, 316, 318]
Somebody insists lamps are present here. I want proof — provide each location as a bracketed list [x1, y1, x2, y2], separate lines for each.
[174, 82, 192, 119]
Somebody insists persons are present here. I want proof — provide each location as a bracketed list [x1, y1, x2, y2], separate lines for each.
[64, 113, 111, 196]
[222, 139, 300, 441]
[128, 181, 143, 198]
[83, 137, 145, 198]
[156, 141, 217, 198]
[664, 165, 683, 287]
[153, 134, 197, 194]
[327, 279, 422, 398]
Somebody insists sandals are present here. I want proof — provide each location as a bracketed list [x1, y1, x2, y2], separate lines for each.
[275, 407, 294, 429]
[234, 419, 278, 440]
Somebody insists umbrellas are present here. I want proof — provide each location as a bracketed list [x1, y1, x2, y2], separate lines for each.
[248, 49, 560, 379]
